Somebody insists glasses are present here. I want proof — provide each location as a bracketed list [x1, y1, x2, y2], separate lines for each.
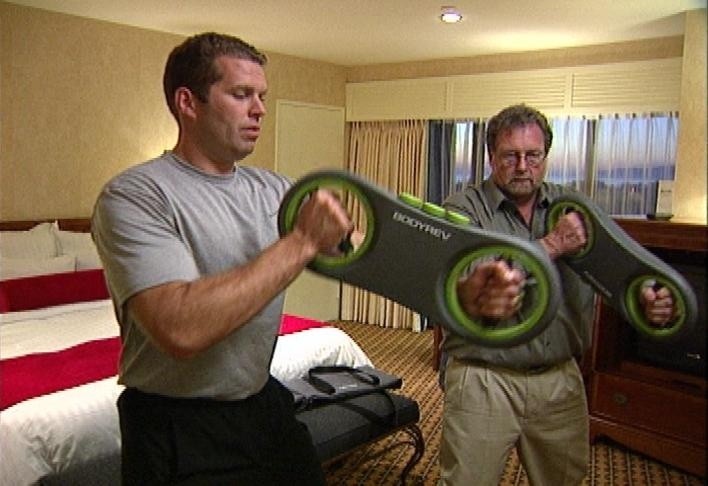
[498, 149, 545, 167]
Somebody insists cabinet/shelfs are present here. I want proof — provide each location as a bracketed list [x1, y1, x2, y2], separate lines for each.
[584, 218, 708, 480]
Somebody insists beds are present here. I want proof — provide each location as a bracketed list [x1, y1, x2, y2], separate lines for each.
[0, 217, 375, 486]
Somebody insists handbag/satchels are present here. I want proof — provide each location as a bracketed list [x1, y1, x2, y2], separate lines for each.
[280, 364, 402, 410]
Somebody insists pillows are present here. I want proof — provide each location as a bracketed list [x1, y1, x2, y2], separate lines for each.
[0, 220, 108, 286]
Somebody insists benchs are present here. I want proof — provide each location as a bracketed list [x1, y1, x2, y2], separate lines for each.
[34, 385, 427, 483]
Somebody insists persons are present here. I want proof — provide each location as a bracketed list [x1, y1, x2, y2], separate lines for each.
[436, 102, 679, 486]
[90, 32, 526, 486]
